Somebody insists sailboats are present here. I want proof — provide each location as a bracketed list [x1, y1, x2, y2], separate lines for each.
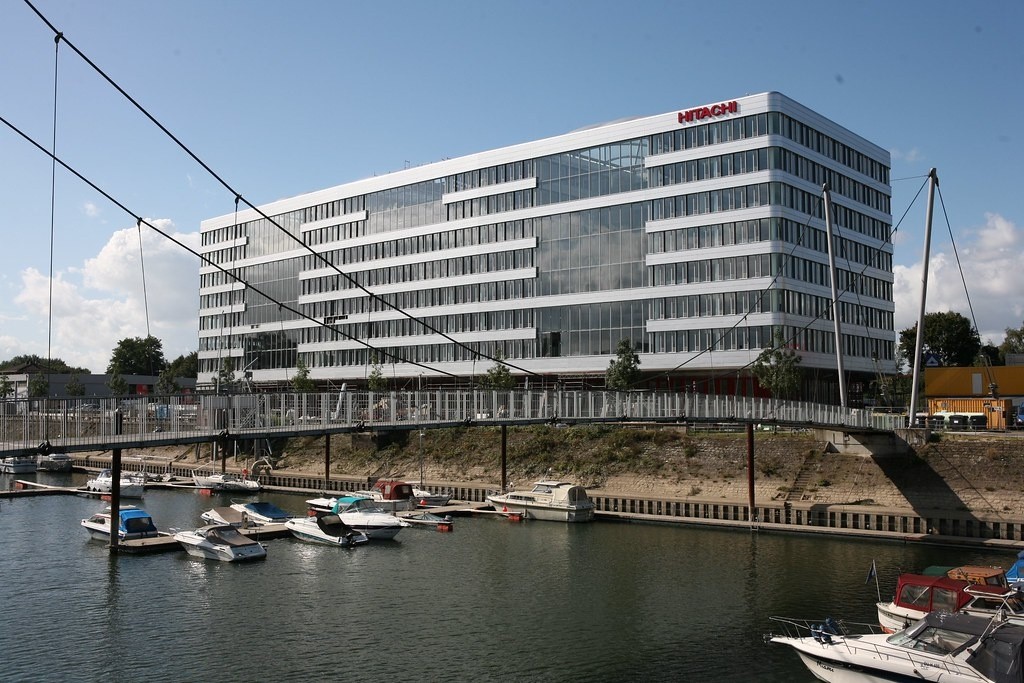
[412, 430, 453, 506]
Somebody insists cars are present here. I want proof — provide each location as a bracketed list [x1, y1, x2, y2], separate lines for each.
[68, 403, 100, 420]
[107, 402, 197, 423]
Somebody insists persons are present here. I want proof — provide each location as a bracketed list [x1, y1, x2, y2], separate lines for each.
[1016, 587, 1022, 601]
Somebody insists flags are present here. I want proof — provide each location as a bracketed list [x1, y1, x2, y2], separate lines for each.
[866, 561, 875, 584]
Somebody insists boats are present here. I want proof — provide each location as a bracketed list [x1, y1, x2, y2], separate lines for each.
[284, 497, 412, 547]
[0, 455, 38, 474]
[172, 525, 267, 562]
[922, 565, 1024, 593]
[487, 481, 596, 523]
[229, 498, 305, 524]
[200, 506, 269, 525]
[1005, 560, 1024, 587]
[305, 491, 406, 517]
[876, 573, 1024, 634]
[80, 505, 170, 543]
[86, 469, 144, 499]
[191, 468, 261, 491]
[400, 511, 455, 532]
[762, 608, 1024, 683]
[40, 453, 76, 472]
